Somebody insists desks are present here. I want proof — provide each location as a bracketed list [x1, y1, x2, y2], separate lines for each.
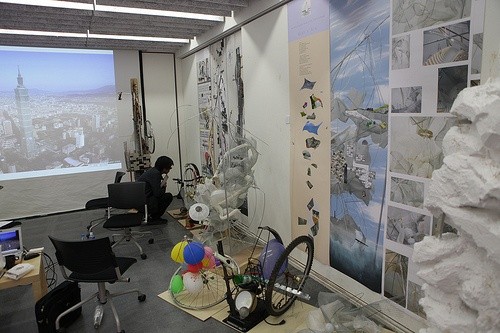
[0, 250, 48, 304]
[0, 221, 23, 251]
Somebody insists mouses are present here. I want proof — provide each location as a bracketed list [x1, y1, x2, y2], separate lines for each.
[24, 253, 39, 260]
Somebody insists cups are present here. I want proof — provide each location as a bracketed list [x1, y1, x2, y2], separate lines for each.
[5, 255, 19, 269]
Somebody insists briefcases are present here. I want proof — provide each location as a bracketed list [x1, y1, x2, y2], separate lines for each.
[34, 280, 82, 333]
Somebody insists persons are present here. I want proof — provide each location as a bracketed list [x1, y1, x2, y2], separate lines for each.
[135, 156, 174, 225]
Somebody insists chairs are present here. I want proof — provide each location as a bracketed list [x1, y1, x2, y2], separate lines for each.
[85, 171, 132, 239]
[102, 182, 155, 260]
[47, 234, 146, 333]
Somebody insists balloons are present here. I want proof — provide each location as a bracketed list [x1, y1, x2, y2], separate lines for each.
[169, 240, 221, 295]
[258, 239, 288, 280]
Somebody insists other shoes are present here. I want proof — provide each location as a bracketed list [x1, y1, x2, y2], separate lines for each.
[149, 217, 168, 225]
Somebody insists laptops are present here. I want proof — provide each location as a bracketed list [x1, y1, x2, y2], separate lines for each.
[0, 227, 22, 266]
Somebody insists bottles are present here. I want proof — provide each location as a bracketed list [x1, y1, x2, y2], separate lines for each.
[89, 232, 96, 240]
